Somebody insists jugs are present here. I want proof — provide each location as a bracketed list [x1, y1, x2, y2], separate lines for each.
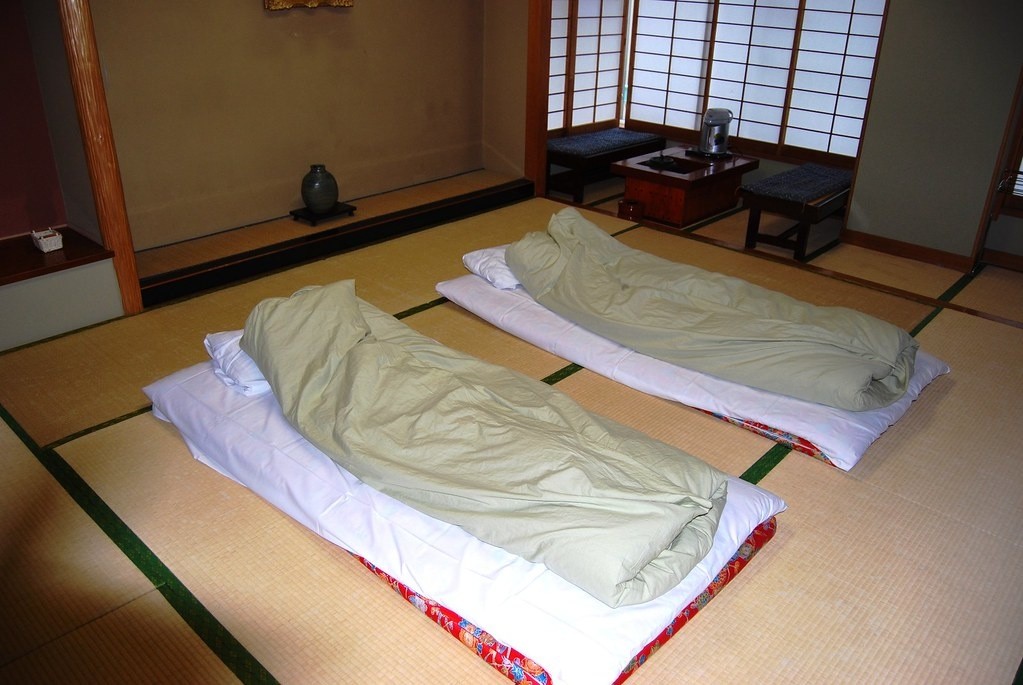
[700, 107, 733, 155]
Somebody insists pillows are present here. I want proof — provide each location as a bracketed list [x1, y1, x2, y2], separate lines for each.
[463, 244, 520, 289]
[204, 330, 271, 395]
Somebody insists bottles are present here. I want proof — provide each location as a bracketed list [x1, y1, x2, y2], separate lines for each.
[301, 164, 338, 211]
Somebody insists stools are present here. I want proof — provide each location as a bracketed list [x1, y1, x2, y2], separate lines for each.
[549, 127, 667, 205]
[736, 163, 853, 263]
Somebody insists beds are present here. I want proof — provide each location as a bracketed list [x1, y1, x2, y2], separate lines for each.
[436, 207, 952, 471]
[143, 281, 790, 685]
